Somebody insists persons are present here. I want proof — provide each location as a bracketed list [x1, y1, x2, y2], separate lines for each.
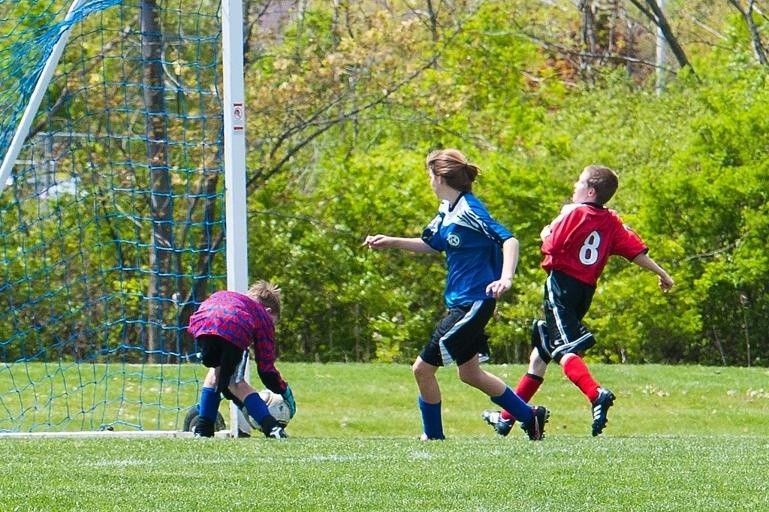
[360, 150, 550, 440]
[481, 166, 674, 436]
[186, 279, 296, 440]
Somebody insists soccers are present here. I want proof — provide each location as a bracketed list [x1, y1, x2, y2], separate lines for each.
[248, 386, 292, 432]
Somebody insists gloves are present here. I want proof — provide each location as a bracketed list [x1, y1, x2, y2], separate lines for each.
[239, 407, 257, 430]
[282, 385, 296, 418]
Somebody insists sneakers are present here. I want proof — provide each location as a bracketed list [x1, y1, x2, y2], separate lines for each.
[194, 418, 214, 439]
[413, 433, 447, 441]
[480, 410, 514, 437]
[521, 405, 551, 442]
[591, 389, 616, 436]
[262, 417, 290, 440]
